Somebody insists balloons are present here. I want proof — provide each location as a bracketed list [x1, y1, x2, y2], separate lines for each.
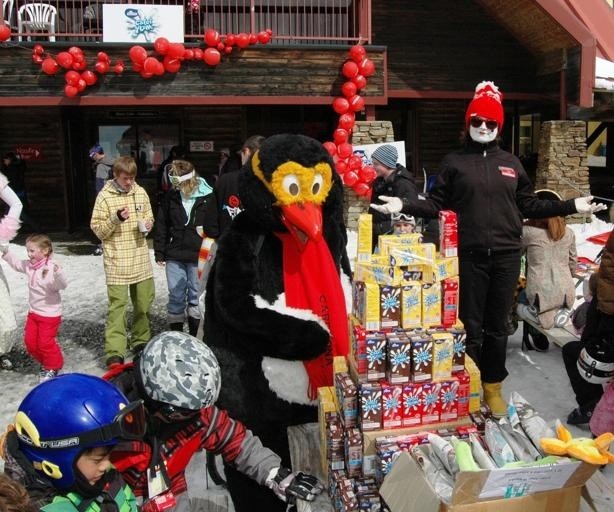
[32, 26, 274, 98]
[0, 25, 11, 41]
[323, 46, 378, 201]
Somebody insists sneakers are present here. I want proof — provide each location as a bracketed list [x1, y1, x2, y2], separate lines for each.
[45, 370, 57, 379]
[566, 405, 592, 425]
[106, 355, 124, 369]
[38, 365, 47, 376]
[0, 357, 13, 369]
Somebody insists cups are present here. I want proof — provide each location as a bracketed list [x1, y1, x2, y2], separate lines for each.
[138, 220, 149, 233]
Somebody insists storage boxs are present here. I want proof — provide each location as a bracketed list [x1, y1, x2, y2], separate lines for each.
[378, 445, 601, 512]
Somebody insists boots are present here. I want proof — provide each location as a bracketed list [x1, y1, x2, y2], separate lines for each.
[483, 383, 508, 418]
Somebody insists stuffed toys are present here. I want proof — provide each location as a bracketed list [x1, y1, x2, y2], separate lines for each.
[199, 139, 352, 512]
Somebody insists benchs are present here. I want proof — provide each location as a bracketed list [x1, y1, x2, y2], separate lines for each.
[524, 310, 583, 349]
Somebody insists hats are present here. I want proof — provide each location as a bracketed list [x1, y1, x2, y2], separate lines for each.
[464, 81, 505, 131]
[371, 144, 398, 168]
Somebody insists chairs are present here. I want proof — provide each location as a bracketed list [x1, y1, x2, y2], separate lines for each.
[17, 3, 102, 42]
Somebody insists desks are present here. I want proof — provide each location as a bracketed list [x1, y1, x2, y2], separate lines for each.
[287, 422, 336, 512]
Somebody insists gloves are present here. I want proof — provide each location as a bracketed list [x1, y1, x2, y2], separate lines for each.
[574, 195, 609, 214]
[265, 467, 328, 505]
[368, 194, 403, 215]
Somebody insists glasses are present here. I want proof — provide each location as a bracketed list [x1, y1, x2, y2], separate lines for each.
[162, 407, 200, 421]
[470, 116, 498, 130]
[111, 399, 148, 441]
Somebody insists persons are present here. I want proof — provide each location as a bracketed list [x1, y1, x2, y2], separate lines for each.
[370, 80, 614, 472]
[0, 135, 267, 512]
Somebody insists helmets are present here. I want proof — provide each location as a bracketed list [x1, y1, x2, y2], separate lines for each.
[135, 329, 222, 411]
[14, 372, 130, 495]
